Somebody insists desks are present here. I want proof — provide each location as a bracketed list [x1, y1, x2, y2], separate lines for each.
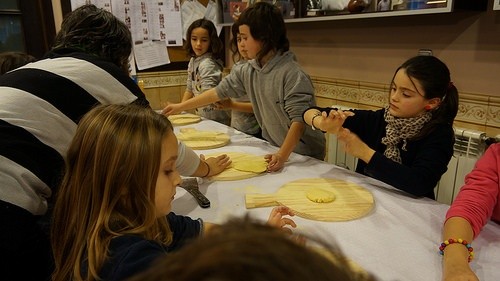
[154, 108, 500, 281]
